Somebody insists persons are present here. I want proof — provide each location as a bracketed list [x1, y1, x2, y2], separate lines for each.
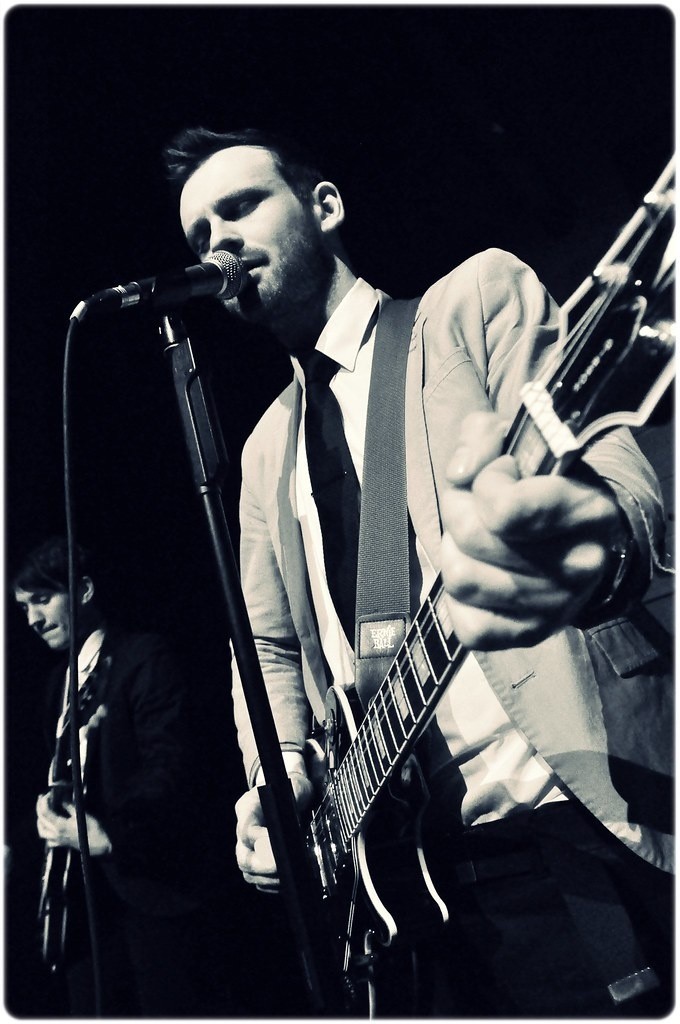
[162, 128, 674, 1017]
[5, 534, 230, 1016]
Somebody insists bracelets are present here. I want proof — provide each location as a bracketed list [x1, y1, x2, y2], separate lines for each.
[599, 539, 635, 604]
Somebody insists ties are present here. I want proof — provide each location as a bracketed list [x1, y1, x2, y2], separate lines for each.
[295, 353, 430, 778]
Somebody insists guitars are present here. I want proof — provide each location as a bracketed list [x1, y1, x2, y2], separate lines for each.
[39, 629, 113, 980]
[301, 150, 676, 1020]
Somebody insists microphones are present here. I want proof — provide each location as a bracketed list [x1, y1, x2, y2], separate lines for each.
[85, 249, 247, 314]
